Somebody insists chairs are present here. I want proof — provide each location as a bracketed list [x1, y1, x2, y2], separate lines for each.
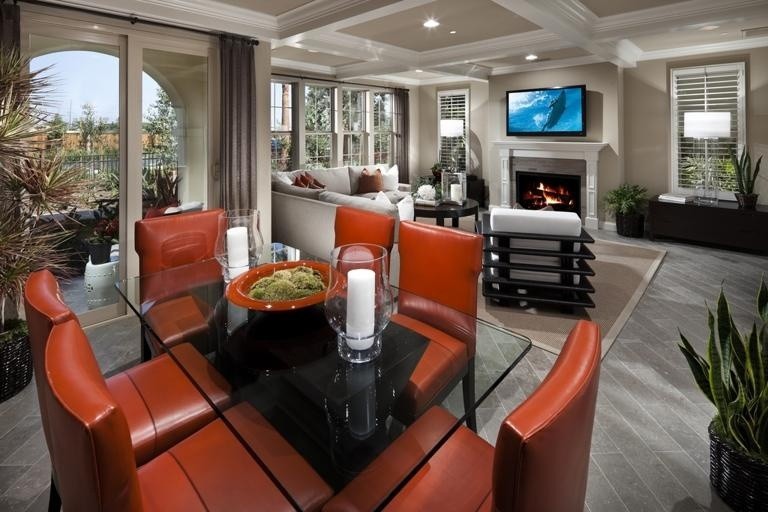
[134, 206, 226, 360]
[322, 318, 605, 510]
[376, 219, 486, 439]
[84, 166, 183, 305]
[470, 206, 597, 314]
[46, 322, 335, 511]
[27, 270, 242, 510]
[320, 204, 392, 312]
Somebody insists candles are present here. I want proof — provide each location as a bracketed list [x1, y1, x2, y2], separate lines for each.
[450, 184, 461, 203]
[226, 227, 249, 276]
[344, 269, 377, 351]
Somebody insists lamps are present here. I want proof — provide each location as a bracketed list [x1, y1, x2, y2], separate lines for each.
[681, 108, 730, 206]
[438, 118, 463, 172]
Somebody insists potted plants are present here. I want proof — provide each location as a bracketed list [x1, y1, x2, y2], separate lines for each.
[600, 180, 646, 237]
[675, 267, 767, 512]
[727, 141, 762, 209]
[430, 163, 441, 180]
[0, 47, 79, 403]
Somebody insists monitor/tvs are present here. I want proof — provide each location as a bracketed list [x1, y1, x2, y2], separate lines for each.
[506, 85, 587, 136]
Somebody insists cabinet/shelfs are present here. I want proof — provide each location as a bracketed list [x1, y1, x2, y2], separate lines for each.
[643, 194, 768, 256]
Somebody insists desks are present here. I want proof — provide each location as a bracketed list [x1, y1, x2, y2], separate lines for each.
[412, 197, 478, 232]
[114, 243, 533, 509]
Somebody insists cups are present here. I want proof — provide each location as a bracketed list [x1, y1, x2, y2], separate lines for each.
[214, 208, 265, 281]
[322, 364, 398, 476]
[324, 243, 394, 363]
[450, 184, 462, 204]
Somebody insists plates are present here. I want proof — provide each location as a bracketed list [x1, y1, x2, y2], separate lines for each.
[225, 261, 343, 313]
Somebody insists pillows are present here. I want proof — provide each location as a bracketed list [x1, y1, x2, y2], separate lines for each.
[356, 168, 383, 194]
[375, 189, 414, 222]
[290, 170, 326, 189]
[375, 162, 398, 191]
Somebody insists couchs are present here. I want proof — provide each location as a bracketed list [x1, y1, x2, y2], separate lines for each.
[270, 160, 412, 303]
